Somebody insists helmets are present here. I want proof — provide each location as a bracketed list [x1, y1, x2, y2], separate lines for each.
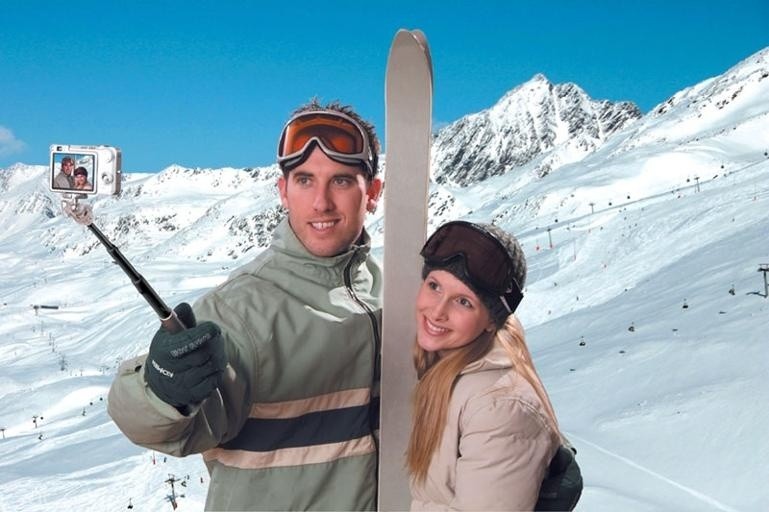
[62, 159, 73, 164]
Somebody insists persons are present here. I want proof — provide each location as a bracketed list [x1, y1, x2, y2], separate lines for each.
[106, 89, 584, 511]
[70, 167, 92, 190]
[401, 216, 574, 511]
[51, 157, 76, 189]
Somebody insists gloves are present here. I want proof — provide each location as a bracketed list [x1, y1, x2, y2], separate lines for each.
[144, 302, 228, 408]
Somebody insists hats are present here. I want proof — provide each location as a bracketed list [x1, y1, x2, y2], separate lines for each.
[419, 220, 527, 329]
[74, 167, 87, 177]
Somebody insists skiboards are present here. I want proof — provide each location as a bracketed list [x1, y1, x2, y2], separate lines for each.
[375, 27, 433, 512]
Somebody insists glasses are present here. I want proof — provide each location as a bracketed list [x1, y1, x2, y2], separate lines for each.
[276, 109, 375, 177]
[420, 221, 524, 314]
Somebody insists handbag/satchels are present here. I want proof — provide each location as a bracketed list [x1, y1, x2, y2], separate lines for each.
[532, 447, 583, 512]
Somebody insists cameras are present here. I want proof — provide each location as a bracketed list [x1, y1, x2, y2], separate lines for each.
[48, 143, 121, 196]
